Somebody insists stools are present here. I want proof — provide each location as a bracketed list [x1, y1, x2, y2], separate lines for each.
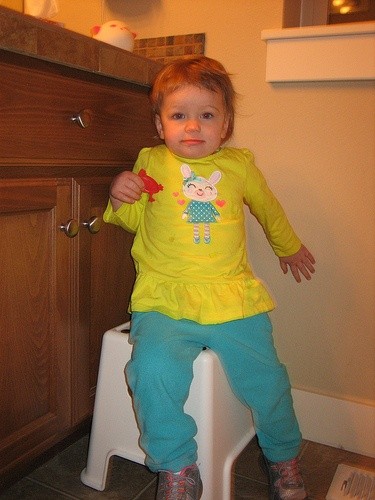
[80, 319, 256, 500]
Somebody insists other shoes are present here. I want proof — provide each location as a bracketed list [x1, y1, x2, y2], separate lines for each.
[262, 455, 308, 500]
[155, 463, 204, 500]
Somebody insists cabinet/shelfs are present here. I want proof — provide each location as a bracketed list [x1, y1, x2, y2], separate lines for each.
[0, 7, 165, 494]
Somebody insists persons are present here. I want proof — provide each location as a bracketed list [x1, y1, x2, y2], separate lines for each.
[104, 53, 316, 500]
